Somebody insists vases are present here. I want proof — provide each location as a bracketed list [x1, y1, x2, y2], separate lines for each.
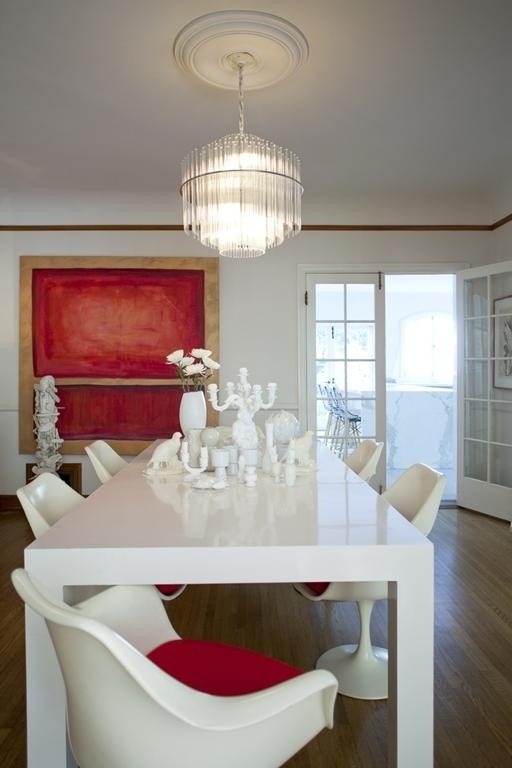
[176, 388, 207, 436]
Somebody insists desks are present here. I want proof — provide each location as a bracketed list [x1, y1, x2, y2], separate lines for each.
[18, 432, 442, 766]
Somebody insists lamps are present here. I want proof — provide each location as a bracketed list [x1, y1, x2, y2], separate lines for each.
[162, 5, 314, 262]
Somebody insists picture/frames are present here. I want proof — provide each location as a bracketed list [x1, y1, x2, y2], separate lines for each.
[491, 293, 512, 393]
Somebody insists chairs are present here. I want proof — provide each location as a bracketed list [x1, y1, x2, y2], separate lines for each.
[81, 438, 131, 485]
[7, 561, 342, 766]
[316, 373, 364, 461]
[293, 459, 449, 706]
[11, 467, 192, 609]
[340, 435, 386, 483]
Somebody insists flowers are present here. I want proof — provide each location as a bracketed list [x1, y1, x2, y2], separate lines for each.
[164, 345, 223, 393]
